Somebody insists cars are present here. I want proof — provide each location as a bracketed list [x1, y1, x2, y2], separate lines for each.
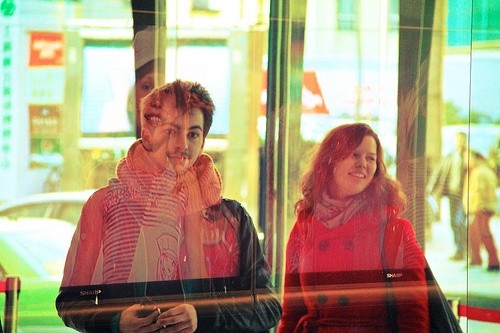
[0, 189, 98, 284]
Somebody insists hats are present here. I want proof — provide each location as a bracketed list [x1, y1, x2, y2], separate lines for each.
[121, 26, 180, 90]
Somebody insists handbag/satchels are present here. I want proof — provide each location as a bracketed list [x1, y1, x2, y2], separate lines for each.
[379, 217, 464, 333]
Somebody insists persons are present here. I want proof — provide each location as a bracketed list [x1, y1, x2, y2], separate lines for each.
[275, 122, 429, 333]
[67, 27, 210, 309]
[426, 130, 472, 260]
[55, 80, 282, 333]
[461, 150, 500, 271]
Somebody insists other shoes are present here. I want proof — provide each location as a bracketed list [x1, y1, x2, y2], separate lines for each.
[449, 254, 462, 260]
[487, 266, 499, 271]
